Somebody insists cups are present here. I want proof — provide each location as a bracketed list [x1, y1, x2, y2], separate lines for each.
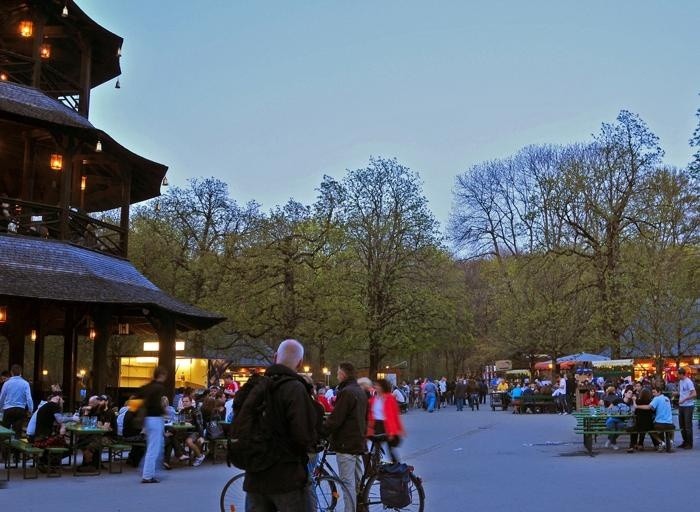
[180, 414, 186, 426]
[173, 414, 178, 425]
[91, 415, 97, 428]
[83, 415, 89, 426]
[590, 404, 627, 415]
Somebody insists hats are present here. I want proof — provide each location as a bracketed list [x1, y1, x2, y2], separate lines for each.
[49, 390, 68, 398]
[96, 395, 110, 402]
[675, 367, 687, 373]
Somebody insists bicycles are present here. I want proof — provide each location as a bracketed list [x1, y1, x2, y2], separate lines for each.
[219, 449, 357, 512]
[312, 431, 425, 511]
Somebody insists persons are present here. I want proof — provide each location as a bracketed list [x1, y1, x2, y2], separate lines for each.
[8, 200, 110, 252]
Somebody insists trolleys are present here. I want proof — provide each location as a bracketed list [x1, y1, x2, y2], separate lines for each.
[490, 389, 508, 411]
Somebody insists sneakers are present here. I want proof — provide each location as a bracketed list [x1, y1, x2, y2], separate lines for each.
[604, 438, 693, 454]
[1, 456, 23, 468]
[161, 452, 206, 470]
[125, 458, 139, 468]
[140, 476, 161, 484]
[34, 456, 101, 473]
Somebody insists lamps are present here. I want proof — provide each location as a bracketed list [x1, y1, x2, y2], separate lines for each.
[20, 17, 33, 37]
[0, 305, 8, 325]
[39, 40, 52, 60]
[50, 150, 65, 173]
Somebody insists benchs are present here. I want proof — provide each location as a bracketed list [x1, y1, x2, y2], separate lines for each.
[511, 387, 576, 413]
[570, 394, 700, 453]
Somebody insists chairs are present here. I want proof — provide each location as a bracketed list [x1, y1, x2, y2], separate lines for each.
[0, 389, 233, 489]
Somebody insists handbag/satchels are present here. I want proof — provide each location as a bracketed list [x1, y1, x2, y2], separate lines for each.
[389, 434, 401, 448]
[379, 462, 412, 509]
[626, 413, 636, 432]
[206, 416, 224, 440]
[330, 414, 351, 446]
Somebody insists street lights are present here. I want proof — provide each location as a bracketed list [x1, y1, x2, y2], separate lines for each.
[386, 360, 408, 370]
[327, 370, 331, 386]
[303, 365, 311, 378]
[322, 366, 328, 385]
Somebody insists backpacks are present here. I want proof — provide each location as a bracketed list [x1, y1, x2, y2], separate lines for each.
[228, 374, 304, 472]
